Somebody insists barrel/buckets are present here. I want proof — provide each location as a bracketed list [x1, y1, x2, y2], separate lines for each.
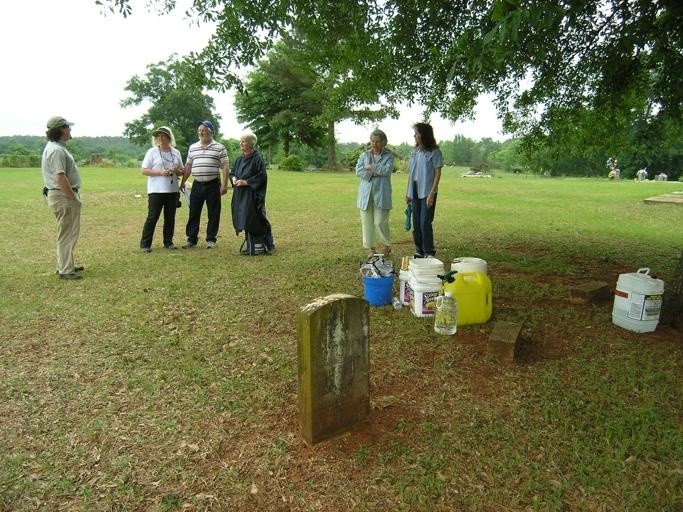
[450, 257, 488, 275]
[359, 266, 395, 306]
[407, 257, 447, 318]
[612, 267, 665, 333]
[398, 270, 412, 305]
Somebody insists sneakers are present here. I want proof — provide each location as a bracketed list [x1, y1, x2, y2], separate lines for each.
[180, 241, 197, 249]
[366, 245, 391, 259]
[240, 249, 249, 255]
[205, 240, 216, 249]
[267, 245, 276, 255]
[140, 247, 152, 254]
[167, 243, 176, 249]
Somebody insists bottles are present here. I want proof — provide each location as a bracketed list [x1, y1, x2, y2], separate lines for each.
[392, 296, 402, 310]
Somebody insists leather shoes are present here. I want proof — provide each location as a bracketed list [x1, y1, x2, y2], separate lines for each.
[54, 265, 84, 275]
[58, 272, 83, 281]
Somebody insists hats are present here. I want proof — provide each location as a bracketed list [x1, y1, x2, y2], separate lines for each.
[46, 115, 74, 132]
[197, 120, 214, 131]
[151, 127, 171, 140]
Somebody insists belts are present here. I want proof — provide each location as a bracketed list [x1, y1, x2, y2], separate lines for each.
[194, 177, 217, 186]
[46, 186, 78, 193]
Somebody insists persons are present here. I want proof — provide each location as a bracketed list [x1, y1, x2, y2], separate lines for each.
[405, 121, 443, 258]
[228, 132, 274, 256]
[179, 120, 230, 249]
[355, 128, 394, 260]
[41, 115, 84, 280]
[139, 125, 185, 253]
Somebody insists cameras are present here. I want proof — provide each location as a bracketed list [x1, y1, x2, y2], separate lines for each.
[167, 171, 174, 176]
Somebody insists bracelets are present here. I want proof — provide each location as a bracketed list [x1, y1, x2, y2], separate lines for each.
[430, 190, 437, 194]
